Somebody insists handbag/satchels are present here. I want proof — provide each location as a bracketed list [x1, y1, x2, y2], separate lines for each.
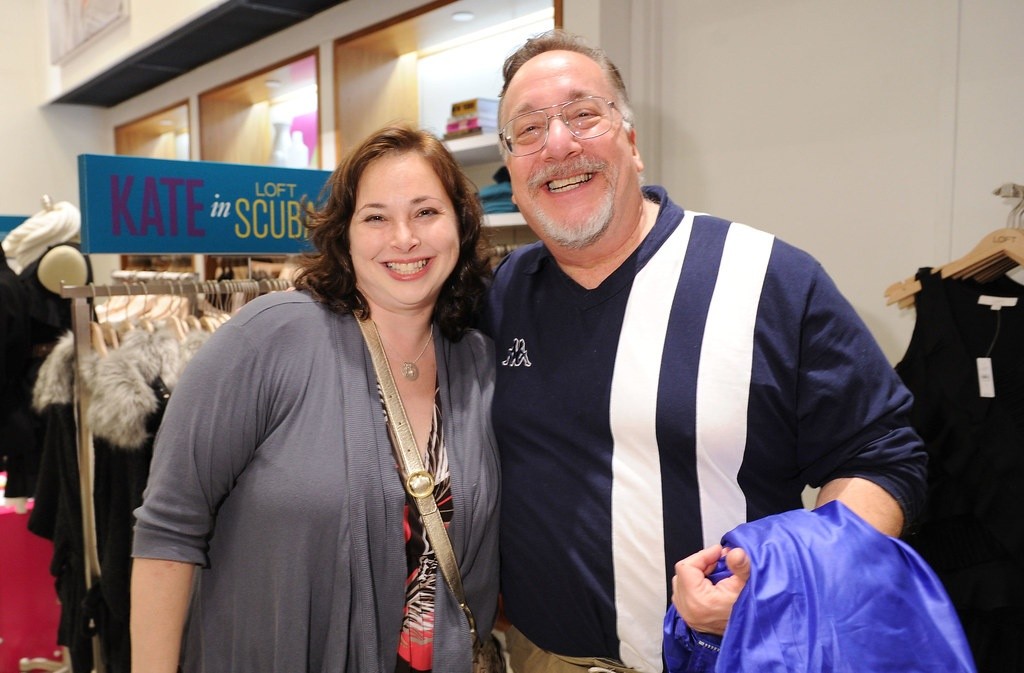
[473, 637, 506, 673]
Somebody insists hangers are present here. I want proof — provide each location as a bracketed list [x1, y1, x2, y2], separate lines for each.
[88, 280, 300, 361]
[883, 183, 1024, 309]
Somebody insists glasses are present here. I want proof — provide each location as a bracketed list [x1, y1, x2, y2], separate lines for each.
[498, 96, 626, 156]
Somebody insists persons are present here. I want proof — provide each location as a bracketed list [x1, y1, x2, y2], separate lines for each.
[130, 125, 502, 673]
[490, 34, 923, 673]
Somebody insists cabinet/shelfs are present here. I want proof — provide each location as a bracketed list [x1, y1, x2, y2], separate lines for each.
[103, 0, 603, 270]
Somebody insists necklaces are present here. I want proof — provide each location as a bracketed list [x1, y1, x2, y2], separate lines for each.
[374, 325, 433, 380]
